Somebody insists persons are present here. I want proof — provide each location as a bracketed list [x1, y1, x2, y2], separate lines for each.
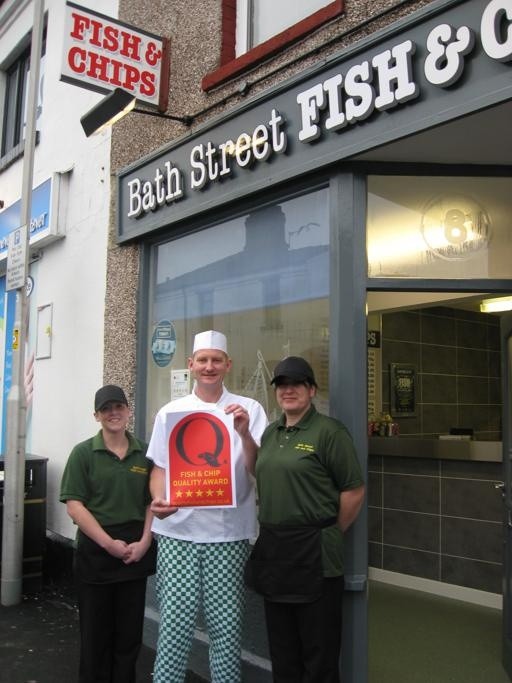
[59, 385, 158, 683]
[255, 356, 365, 683]
[146, 330, 270, 683]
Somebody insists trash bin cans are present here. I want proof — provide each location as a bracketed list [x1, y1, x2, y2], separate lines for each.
[0, 452, 49, 595]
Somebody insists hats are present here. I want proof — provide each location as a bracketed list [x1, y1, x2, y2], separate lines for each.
[192, 331, 228, 356]
[95, 385, 127, 410]
[271, 357, 313, 385]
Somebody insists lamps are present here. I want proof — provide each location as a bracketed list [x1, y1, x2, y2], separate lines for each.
[80, 88, 193, 139]
[480, 296, 512, 312]
